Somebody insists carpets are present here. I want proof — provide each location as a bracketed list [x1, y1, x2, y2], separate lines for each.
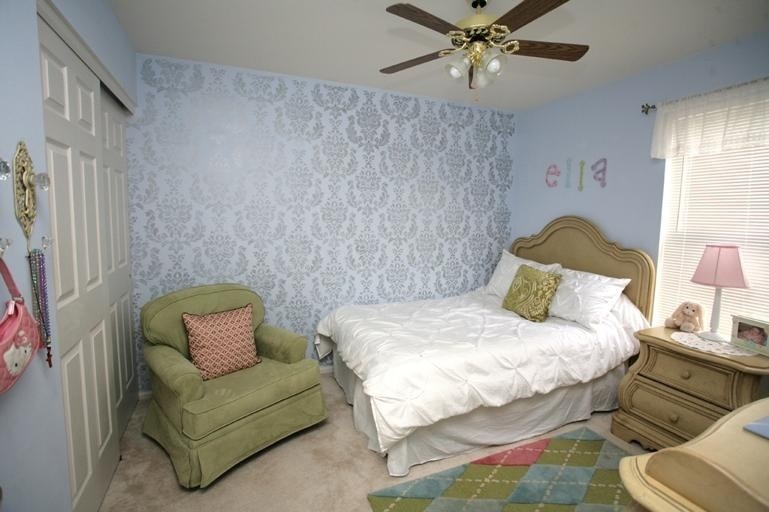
[366, 428, 650, 512]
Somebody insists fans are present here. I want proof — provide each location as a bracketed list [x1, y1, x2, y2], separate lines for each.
[379, 0, 589, 74]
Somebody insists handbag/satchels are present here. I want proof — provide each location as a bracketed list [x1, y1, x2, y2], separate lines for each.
[2, 255, 42, 396]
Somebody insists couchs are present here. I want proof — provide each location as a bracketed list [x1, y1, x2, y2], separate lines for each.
[138, 283, 328, 490]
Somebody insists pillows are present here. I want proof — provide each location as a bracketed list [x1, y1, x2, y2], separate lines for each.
[484, 248, 556, 320]
[548, 268, 631, 332]
[502, 266, 564, 323]
[182, 304, 262, 381]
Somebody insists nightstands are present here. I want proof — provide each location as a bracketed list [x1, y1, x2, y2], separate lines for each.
[610, 323, 769, 452]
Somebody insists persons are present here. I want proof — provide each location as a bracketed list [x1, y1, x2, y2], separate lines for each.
[741, 326, 764, 346]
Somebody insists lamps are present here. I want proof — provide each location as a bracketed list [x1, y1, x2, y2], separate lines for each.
[439, 4, 521, 91]
[693, 243, 749, 345]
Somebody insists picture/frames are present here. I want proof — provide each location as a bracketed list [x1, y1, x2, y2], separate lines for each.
[731, 315, 769, 361]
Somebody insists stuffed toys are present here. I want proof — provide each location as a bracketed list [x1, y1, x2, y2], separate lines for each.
[664, 301, 705, 333]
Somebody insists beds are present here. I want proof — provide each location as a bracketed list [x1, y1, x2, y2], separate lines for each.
[318, 213, 654, 476]
